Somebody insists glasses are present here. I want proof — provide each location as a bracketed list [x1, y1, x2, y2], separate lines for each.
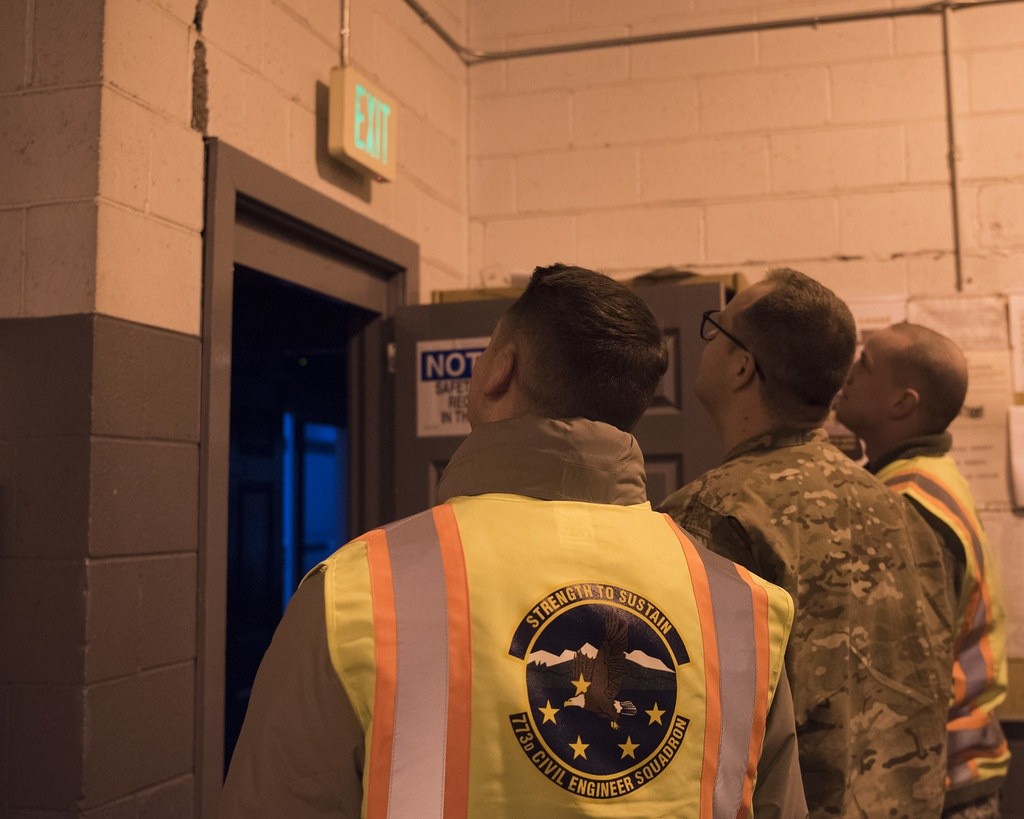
[700, 310, 766, 382]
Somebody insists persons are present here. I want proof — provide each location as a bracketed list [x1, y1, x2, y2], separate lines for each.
[652, 266, 959, 819]
[832, 322, 1013, 819]
[207, 261, 813, 819]
